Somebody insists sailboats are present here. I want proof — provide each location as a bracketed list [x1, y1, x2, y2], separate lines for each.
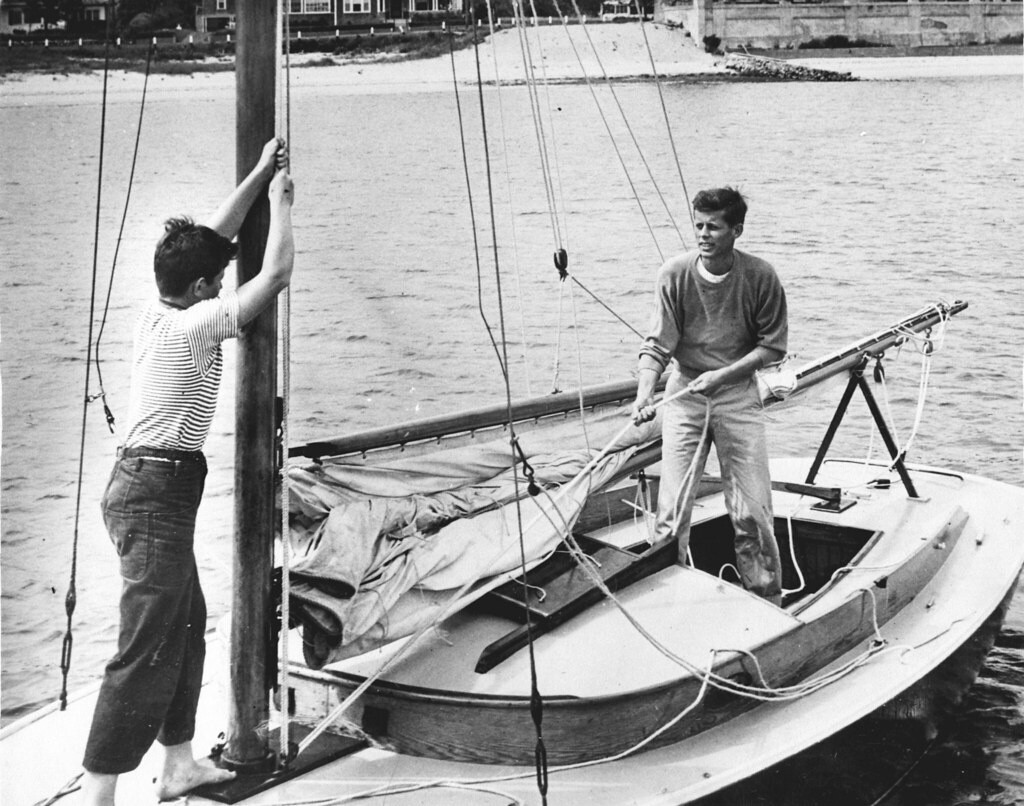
[1, 0, 1022, 806]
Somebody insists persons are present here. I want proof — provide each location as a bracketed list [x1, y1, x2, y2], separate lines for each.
[630, 188, 787, 608]
[77, 136, 296, 806]
